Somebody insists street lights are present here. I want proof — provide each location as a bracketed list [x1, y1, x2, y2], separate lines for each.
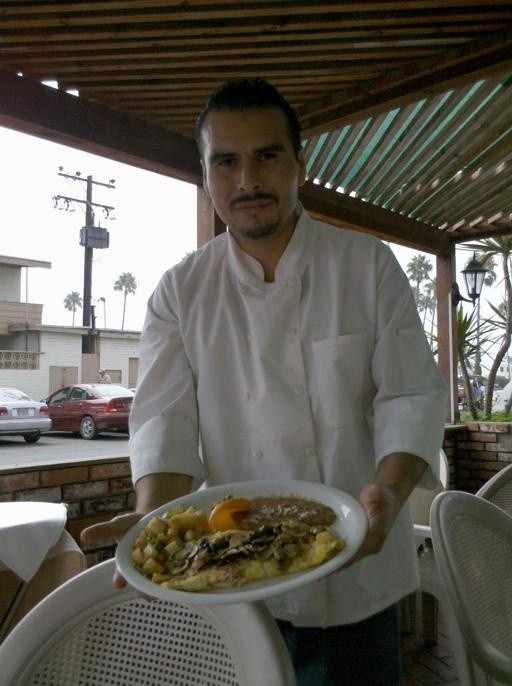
[100, 298, 106, 328]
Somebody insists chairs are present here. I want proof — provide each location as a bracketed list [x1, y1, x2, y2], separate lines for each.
[410, 448, 511, 684]
[0, 556, 297, 686]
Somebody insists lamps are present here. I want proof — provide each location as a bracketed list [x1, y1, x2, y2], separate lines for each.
[450, 249, 490, 309]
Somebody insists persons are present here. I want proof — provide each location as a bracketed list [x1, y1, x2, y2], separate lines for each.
[80, 74, 450, 683]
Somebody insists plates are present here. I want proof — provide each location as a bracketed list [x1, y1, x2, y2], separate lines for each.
[112, 476, 370, 608]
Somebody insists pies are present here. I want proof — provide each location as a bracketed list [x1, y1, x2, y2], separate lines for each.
[154, 520, 345, 591]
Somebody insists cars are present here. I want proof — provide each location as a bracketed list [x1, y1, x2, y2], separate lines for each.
[41, 382, 135, 440]
[0, 387, 52, 442]
